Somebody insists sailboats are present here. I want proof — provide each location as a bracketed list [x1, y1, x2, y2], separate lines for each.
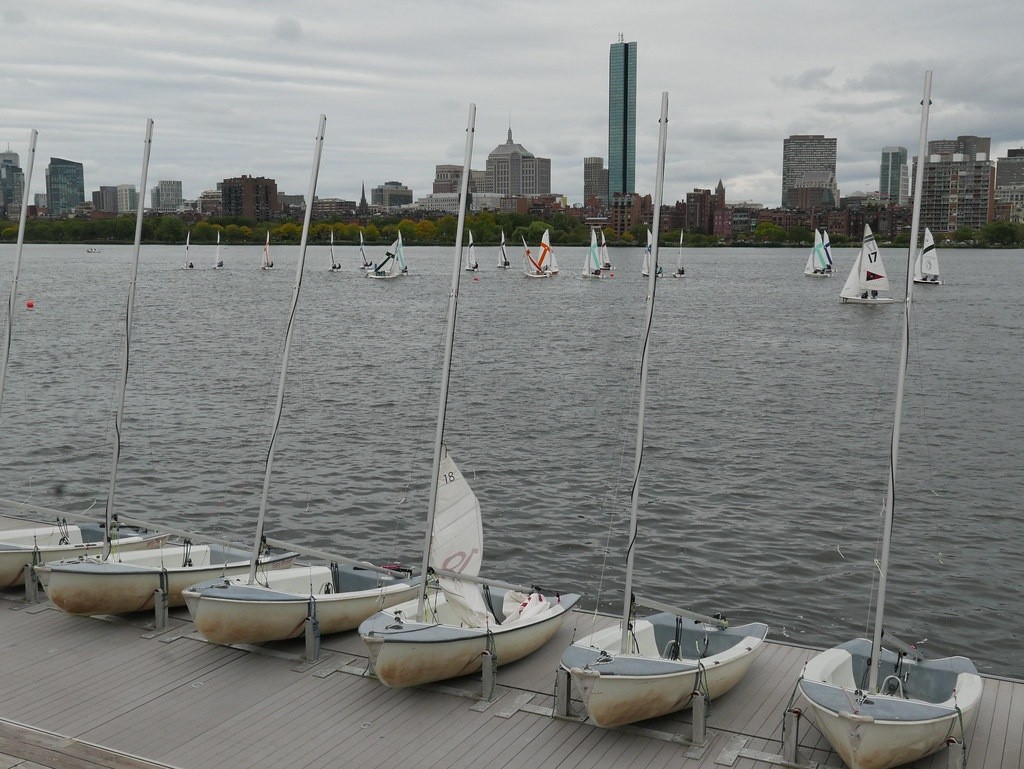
[181, 230, 196, 270]
[356, 230, 376, 270]
[330, 229, 345, 271]
[838, 223, 895, 304]
[0, 128, 168, 594]
[496, 230, 511, 269]
[32, 120, 297, 618]
[558, 91, 768, 729]
[803, 228, 833, 280]
[911, 228, 944, 285]
[794, 64, 981, 768]
[261, 230, 275, 270]
[355, 103, 581, 691]
[181, 109, 423, 647]
[580, 230, 605, 279]
[213, 231, 226, 270]
[521, 235, 550, 279]
[539, 229, 560, 274]
[641, 228, 664, 276]
[600, 231, 614, 272]
[822, 229, 837, 274]
[466, 231, 479, 272]
[366, 240, 399, 278]
[397, 228, 409, 275]
[672, 228, 687, 279]
